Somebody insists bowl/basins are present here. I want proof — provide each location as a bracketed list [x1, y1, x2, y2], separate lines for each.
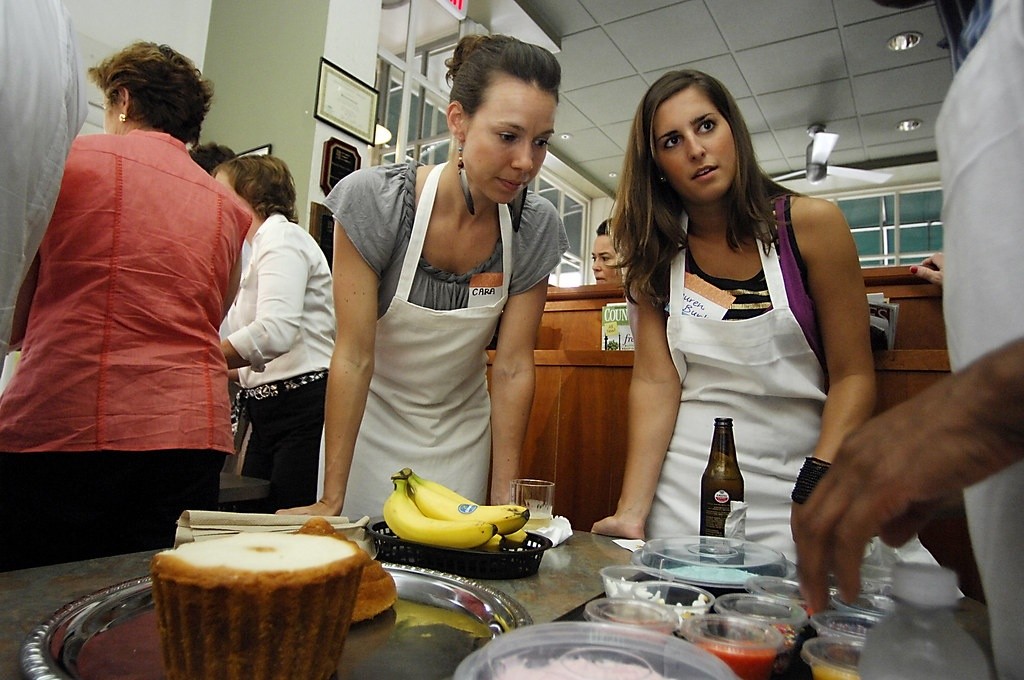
[453, 621, 742, 680]
[583, 534, 898, 680]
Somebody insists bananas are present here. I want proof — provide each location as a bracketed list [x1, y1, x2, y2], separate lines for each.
[384, 466, 532, 553]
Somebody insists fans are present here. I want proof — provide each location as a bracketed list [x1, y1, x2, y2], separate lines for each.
[770, 123, 894, 185]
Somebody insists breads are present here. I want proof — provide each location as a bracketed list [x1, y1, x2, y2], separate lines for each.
[149, 532, 368, 680]
[278, 515, 398, 623]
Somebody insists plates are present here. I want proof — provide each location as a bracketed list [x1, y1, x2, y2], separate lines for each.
[21, 562, 533, 679]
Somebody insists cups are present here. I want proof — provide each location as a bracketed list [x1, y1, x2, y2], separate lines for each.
[508, 478, 554, 531]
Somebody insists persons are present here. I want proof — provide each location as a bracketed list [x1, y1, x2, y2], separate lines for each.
[188, 141, 238, 177]
[789, 0, 1024, 680]
[0, 0, 90, 379]
[209, 154, 336, 513]
[592, 219, 629, 286]
[275, 34, 571, 516]
[589, 69, 965, 601]
[910, 252, 943, 283]
[0, 42, 255, 575]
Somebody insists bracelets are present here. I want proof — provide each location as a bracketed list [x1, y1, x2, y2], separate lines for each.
[791, 457, 832, 505]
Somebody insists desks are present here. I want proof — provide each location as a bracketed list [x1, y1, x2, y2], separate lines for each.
[0, 528, 997, 680]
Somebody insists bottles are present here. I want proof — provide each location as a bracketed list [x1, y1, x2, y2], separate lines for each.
[857, 563, 992, 679]
[698, 417, 745, 564]
